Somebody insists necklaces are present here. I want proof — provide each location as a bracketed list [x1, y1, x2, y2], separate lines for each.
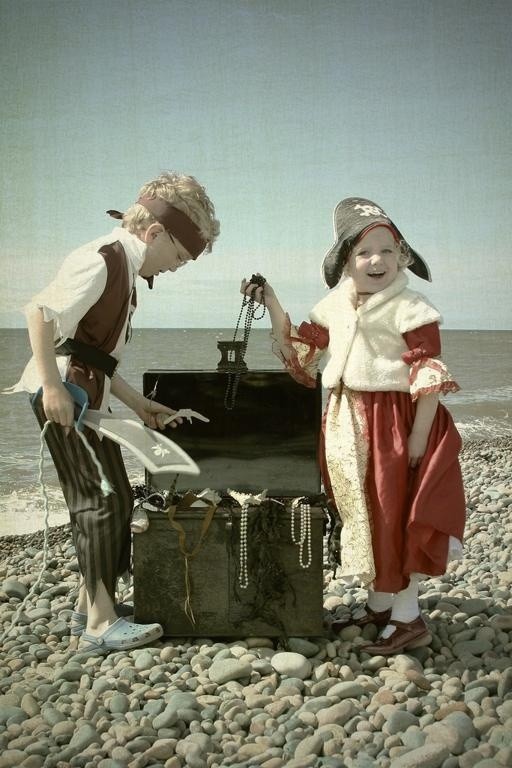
[289, 499, 308, 546]
[224, 272, 267, 413]
[238, 503, 250, 591]
[298, 504, 313, 569]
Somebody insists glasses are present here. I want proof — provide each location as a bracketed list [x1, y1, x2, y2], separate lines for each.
[152, 221, 187, 268]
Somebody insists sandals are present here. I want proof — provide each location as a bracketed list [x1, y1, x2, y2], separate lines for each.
[77, 615, 164, 656]
[69, 602, 135, 636]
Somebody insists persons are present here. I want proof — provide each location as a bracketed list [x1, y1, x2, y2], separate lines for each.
[236, 195, 470, 659]
[0, 168, 223, 655]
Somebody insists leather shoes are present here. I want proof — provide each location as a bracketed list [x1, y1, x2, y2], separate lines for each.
[359, 614, 429, 655]
[329, 602, 393, 635]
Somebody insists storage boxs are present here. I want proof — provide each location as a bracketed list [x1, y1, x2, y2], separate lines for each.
[129, 339, 329, 642]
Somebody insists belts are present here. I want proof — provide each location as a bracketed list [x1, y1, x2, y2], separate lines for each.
[52, 336, 121, 381]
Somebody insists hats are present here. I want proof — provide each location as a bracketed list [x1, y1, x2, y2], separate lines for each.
[320, 196, 433, 293]
